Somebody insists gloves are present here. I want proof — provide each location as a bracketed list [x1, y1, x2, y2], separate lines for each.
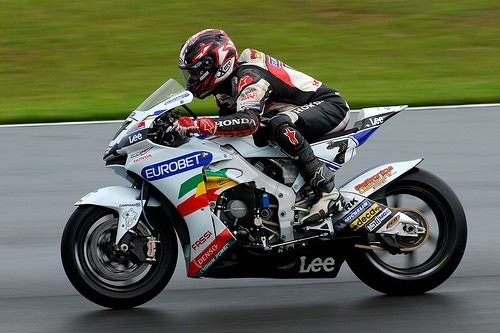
[174, 116, 218, 138]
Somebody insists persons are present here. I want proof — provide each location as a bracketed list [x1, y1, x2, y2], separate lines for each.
[166, 28, 351, 226]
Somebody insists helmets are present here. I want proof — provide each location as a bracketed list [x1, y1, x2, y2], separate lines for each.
[179, 27, 238, 99]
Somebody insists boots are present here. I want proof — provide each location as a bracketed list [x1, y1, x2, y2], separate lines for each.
[300, 161, 346, 226]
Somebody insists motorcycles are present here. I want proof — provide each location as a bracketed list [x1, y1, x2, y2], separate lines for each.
[61, 78, 468, 309]
